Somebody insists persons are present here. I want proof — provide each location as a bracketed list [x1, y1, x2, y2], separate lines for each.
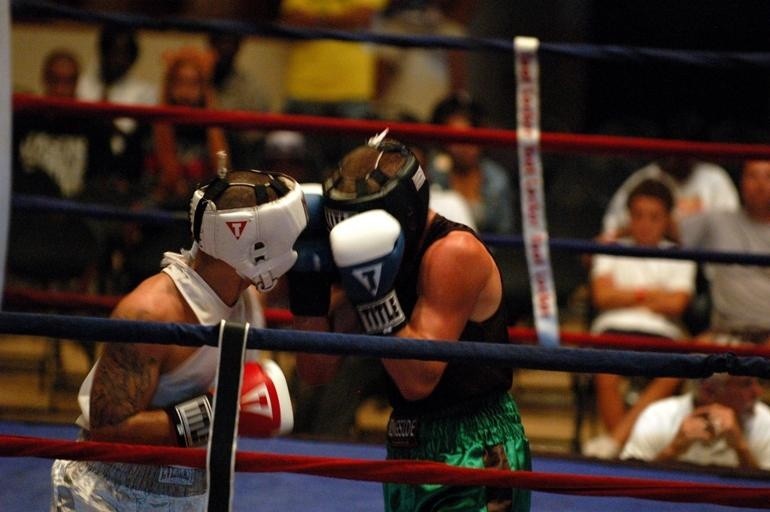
[286, 127, 531, 512]
[1, 1, 769, 470]
[46, 168, 311, 511]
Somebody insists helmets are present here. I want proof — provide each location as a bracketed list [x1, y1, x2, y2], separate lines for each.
[192, 174, 307, 288]
[323, 149, 428, 257]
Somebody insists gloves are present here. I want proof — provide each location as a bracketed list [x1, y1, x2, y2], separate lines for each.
[288, 208, 408, 336]
[167, 358, 296, 449]
